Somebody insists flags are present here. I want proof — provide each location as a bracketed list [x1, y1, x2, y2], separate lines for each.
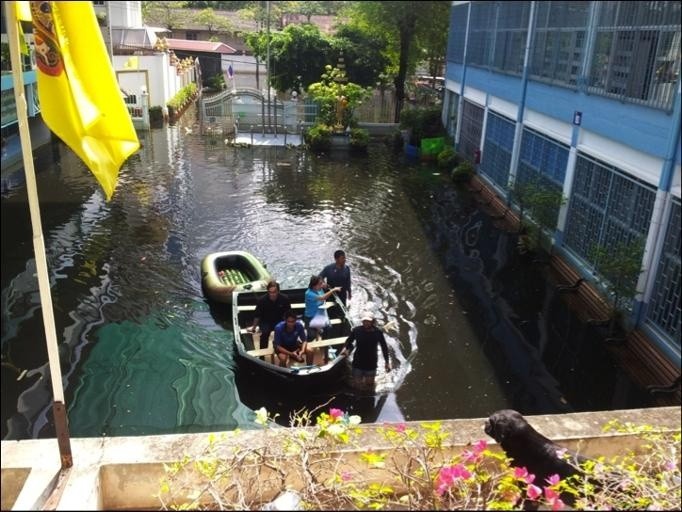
[12, 1, 141, 203]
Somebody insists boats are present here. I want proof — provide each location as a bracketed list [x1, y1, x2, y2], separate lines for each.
[198, 251, 277, 302]
[229, 281, 358, 386]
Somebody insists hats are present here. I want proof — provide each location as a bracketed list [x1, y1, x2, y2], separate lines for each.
[359, 311, 376, 322]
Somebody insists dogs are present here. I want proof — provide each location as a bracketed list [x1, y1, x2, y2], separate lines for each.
[483, 408, 640, 512]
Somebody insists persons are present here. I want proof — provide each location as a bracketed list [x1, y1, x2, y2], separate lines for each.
[318, 250, 353, 307]
[274, 309, 314, 369]
[304, 275, 344, 341]
[246, 281, 292, 361]
[341, 311, 391, 384]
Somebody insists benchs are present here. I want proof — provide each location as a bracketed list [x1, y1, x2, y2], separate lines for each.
[534, 255, 681, 405]
[236, 301, 350, 358]
[464, 173, 528, 239]
